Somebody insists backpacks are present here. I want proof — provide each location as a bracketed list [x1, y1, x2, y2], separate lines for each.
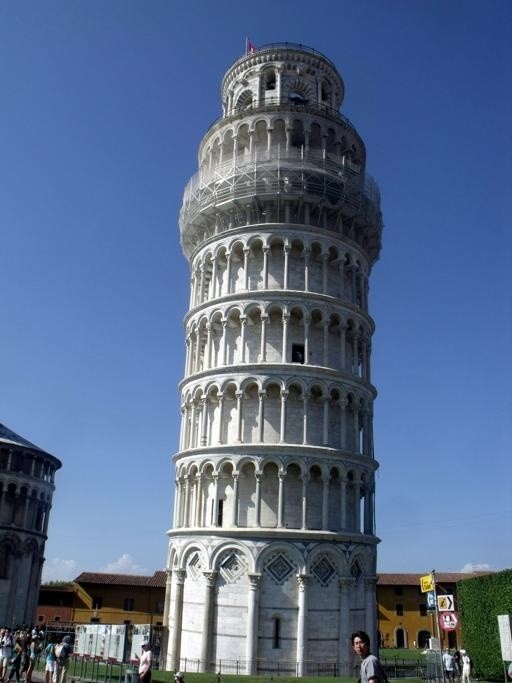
[54, 642, 66, 659]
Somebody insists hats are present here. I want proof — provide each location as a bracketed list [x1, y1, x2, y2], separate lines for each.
[139, 641, 149, 646]
[173, 672, 184, 678]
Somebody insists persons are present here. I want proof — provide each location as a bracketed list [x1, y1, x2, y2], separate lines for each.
[442, 648, 472, 683]
[0, 622, 74, 683]
[349, 630, 385, 683]
[173, 672, 185, 683]
[133, 640, 154, 682]
